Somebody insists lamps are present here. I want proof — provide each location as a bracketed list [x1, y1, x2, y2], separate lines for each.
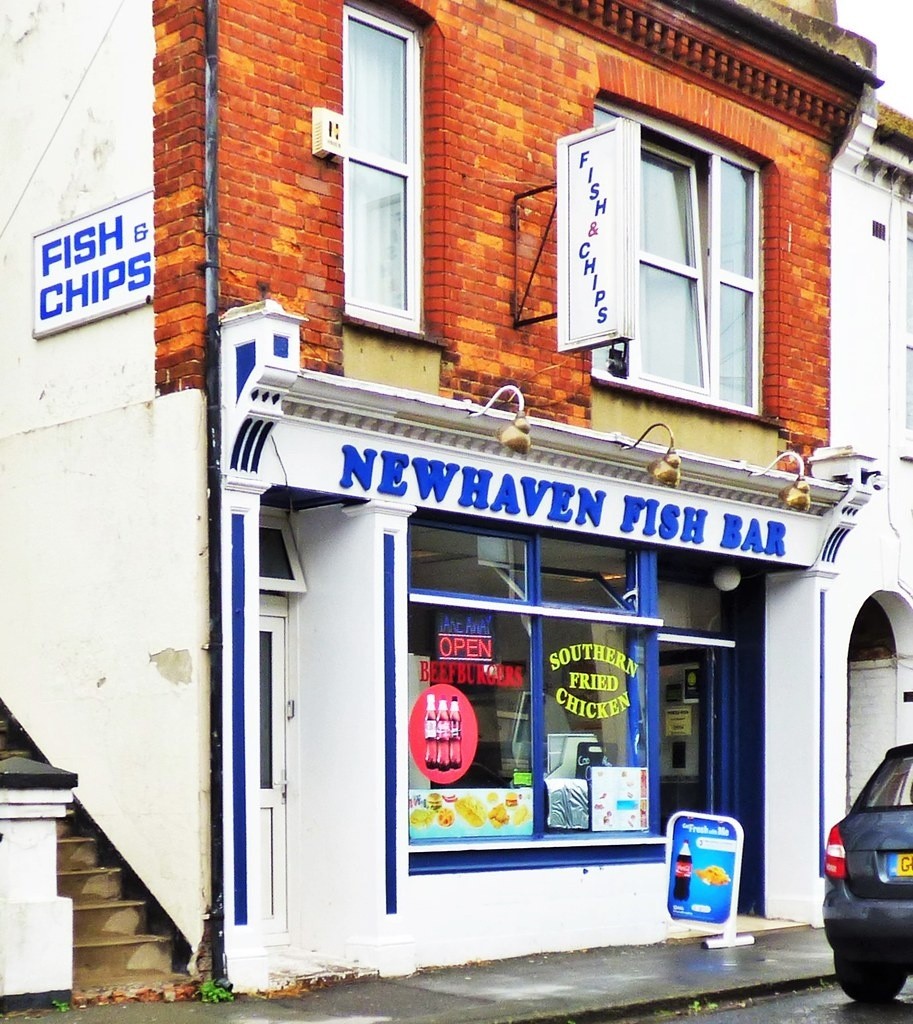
[621, 423, 681, 488]
[749, 451, 810, 512]
[468, 385, 531, 455]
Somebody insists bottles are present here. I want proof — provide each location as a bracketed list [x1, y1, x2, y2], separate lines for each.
[448, 695, 462, 768]
[435, 694, 451, 771]
[424, 693, 438, 770]
[672, 839, 692, 902]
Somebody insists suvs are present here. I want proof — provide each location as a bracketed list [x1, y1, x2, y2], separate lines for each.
[822, 742, 913, 1001]
